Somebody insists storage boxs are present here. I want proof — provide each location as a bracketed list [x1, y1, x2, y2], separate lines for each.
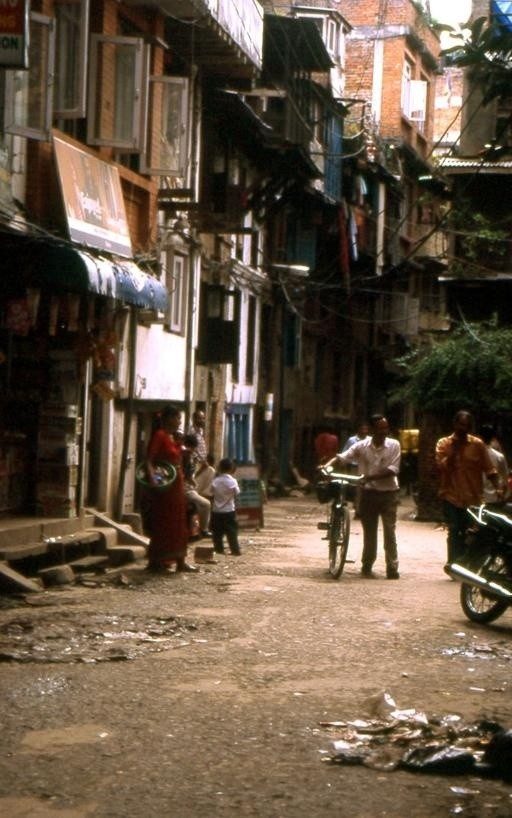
[33, 377, 84, 518]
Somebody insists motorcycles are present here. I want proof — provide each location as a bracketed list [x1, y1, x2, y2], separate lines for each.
[444, 504, 512, 623]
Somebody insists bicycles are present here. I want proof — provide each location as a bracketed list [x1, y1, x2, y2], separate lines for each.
[319, 466, 365, 579]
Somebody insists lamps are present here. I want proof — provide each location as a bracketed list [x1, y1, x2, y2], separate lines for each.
[247, 261, 311, 280]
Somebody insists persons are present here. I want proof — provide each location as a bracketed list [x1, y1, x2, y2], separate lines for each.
[433, 409, 507, 582]
[208, 458, 242, 555]
[342, 421, 372, 520]
[315, 413, 402, 580]
[187, 409, 215, 498]
[478, 423, 508, 502]
[140, 405, 200, 575]
[173, 431, 213, 540]
[316, 420, 339, 463]
[182, 431, 208, 487]
